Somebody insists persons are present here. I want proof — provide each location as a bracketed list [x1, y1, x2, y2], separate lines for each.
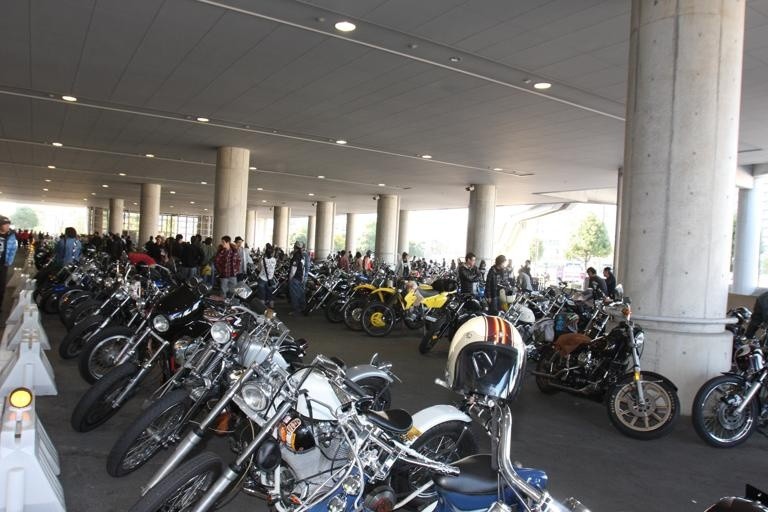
[257, 240, 311, 315]
[143, 233, 259, 304]
[394, 252, 456, 279]
[457, 252, 533, 316]
[333, 250, 374, 279]
[583, 267, 616, 299]
[1, 216, 135, 307]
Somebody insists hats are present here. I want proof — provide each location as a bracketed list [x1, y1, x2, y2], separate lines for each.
[0, 217, 10, 224]
[233, 237, 244, 241]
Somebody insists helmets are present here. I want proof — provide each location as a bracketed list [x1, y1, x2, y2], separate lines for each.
[445, 315, 526, 399]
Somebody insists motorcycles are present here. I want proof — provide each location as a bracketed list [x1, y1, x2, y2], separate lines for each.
[33, 234, 767, 512]
